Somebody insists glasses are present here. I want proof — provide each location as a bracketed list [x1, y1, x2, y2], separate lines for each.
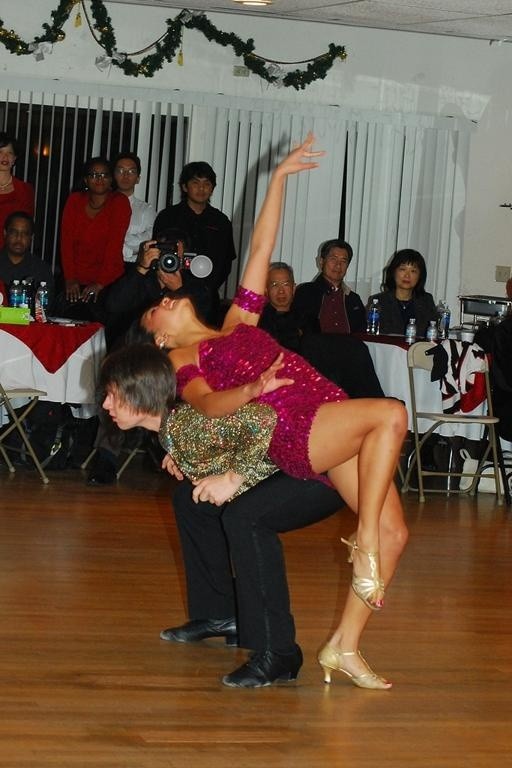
[87, 170, 112, 179]
[113, 167, 138, 175]
[182, 181, 214, 188]
[10, 229, 28, 237]
[269, 280, 293, 289]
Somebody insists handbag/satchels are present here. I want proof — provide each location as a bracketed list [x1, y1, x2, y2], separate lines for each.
[459, 447, 506, 495]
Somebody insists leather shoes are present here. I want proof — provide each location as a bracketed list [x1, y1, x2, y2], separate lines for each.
[160, 619, 238, 647]
[224, 644, 303, 688]
[143, 443, 167, 471]
[87, 458, 116, 486]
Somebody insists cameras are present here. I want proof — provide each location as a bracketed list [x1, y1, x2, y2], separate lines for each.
[138, 242, 179, 273]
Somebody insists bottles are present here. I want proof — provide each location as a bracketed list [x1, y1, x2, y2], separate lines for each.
[437, 304, 451, 339]
[34, 281, 49, 323]
[366, 299, 381, 336]
[426, 320, 437, 342]
[405, 318, 417, 345]
[9, 280, 22, 309]
[20, 279, 32, 308]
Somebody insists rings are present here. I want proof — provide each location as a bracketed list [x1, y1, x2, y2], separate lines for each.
[88, 292, 94, 295]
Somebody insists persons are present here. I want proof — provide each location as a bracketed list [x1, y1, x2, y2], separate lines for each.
[0, 210, 56, 304]
[152, 161, 238, 326]
[98, 342, 347, 690]
[124, 131, 411, 690]
[257, 261, 406, 407]
[366, 248, 438, 338]
[0, 131, 36, 306]
[86, 227, 212, 486]
[59, 156, 132, 303]
[290, 239, 367, 336]
[111, 151, 156, 271]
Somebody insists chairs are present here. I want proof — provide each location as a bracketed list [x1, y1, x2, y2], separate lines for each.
[401, 338, 504, 506]
[0, 382, 50, 485]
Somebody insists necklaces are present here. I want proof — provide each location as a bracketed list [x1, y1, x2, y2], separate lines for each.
[88, 202, 105, 210]
[0, 176, 12, 191]
[89, 197, 104, 210]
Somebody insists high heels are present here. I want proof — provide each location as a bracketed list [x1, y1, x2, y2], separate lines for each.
[316, 643, 392, 690]
[340, 532, 386, 610]
